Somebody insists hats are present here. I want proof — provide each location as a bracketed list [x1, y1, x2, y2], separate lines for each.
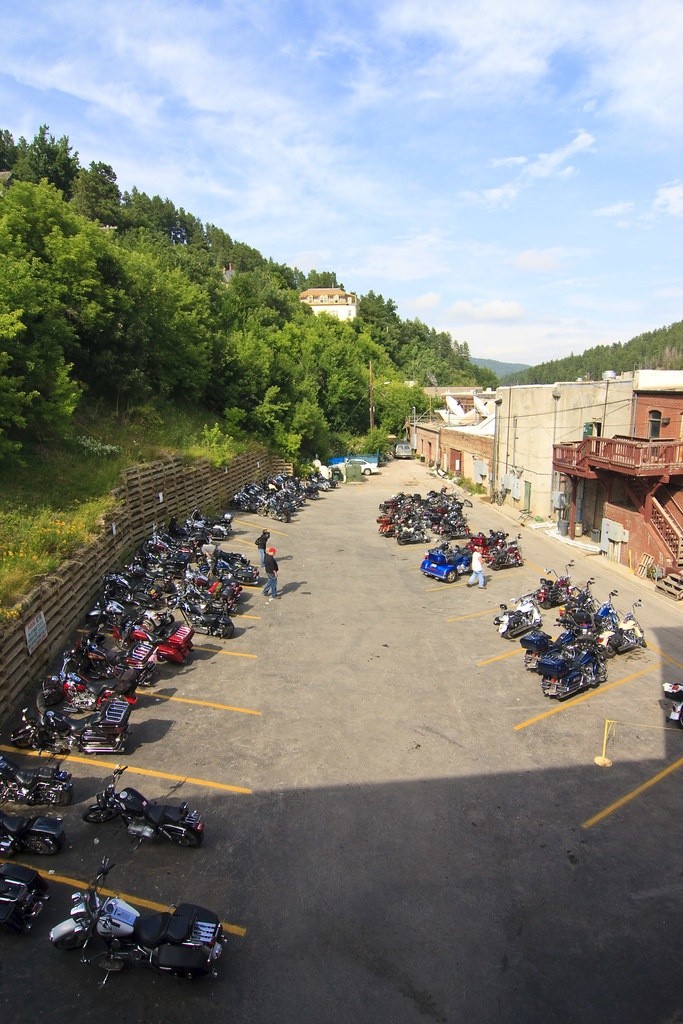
[269, 548, 276, 553]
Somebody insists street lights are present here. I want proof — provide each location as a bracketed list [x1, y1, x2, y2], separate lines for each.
[369, 382, 391, 429]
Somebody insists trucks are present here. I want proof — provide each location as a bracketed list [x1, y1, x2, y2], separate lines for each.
[392, 444, 413, 460]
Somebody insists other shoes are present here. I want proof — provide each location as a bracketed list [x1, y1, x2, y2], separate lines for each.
[466, 584, 471, 587]
[479, 586, 487, 589]
[273, 595, 280, 599]
[262, 591, 270, 596]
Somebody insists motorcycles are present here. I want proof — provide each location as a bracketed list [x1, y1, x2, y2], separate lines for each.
[0, 753, 73, 807]
[520, 618, 608, 702]
[662, 682, 683, 729]
[230, 473, 338, 523]
[48, 856, 229, 989]
[63, 624, 162, 687]
[83, 766, 204, 852]
[420, 530, 524, 583]
[9, 683, 132, 761]
[0, 862, 51, 932]
[533, 560, 575, 610]
[376, 487, 473, 546]
[87, 507, 260, 666]
[40, 658, 140, 713]
[553, 577, 647, 658]
[492, 592, 543, 639]
[0, 796, 66, 858]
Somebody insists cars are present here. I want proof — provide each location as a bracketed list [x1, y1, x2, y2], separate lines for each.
[328, 460, 379, 475]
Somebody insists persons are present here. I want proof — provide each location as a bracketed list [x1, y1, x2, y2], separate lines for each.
[255, 529, 271, 570]
[466, 547, 487, 589]
[262, 547, 281, 599]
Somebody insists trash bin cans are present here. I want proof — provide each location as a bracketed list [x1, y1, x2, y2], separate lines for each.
[333, 468, 340, 480]
[346, 461, 362, 483]
[558, 520, 569, 536]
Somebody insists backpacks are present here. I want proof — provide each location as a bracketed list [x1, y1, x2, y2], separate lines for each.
[255, 537, 262, 545]
